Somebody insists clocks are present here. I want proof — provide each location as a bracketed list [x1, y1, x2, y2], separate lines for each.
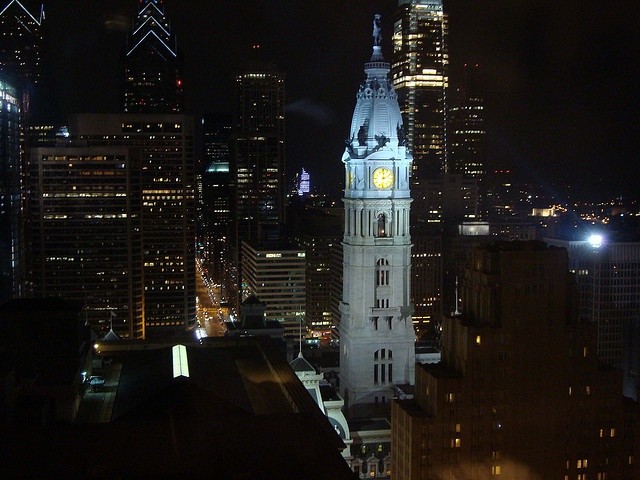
[372, 166, 395, 190]
